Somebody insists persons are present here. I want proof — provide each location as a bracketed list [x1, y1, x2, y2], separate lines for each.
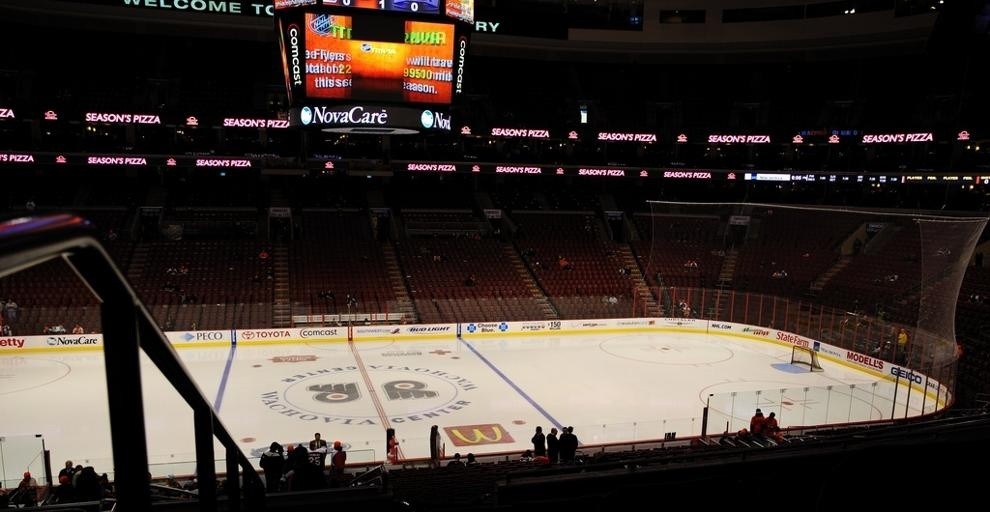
[1, 176, 990, 509]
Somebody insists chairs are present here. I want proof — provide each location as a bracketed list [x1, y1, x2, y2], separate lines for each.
[1, 424, 809, 512]
[812, 338, 989, 510]
[0, 185, 989, 338]
